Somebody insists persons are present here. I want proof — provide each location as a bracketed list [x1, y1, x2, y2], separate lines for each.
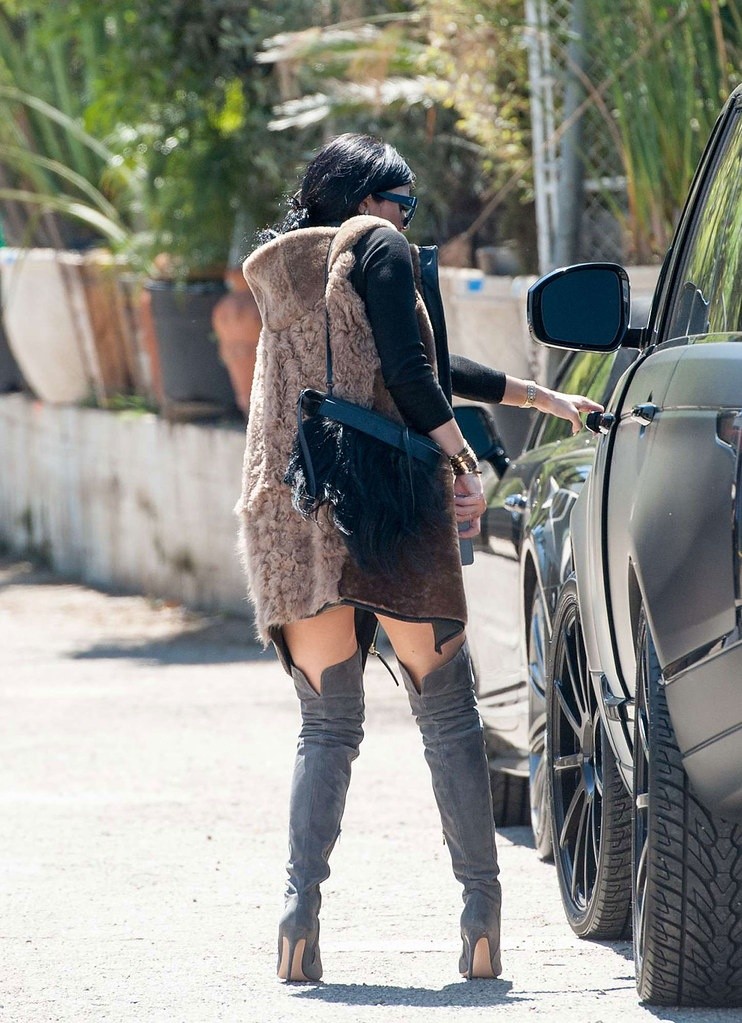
[235, 130, 606, 981]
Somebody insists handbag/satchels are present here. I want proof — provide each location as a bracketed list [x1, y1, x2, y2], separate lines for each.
[281, 384, 451, 567]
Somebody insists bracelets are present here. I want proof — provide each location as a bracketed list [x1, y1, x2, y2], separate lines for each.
[448, 441, 482, 476]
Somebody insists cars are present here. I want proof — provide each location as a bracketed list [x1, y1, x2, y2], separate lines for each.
[453, 77, 742, 1016]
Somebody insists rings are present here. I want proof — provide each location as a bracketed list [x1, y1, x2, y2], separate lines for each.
[469, 514, 473, 520]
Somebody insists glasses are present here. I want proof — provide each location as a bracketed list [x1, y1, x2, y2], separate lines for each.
[374, 188, 418, 226]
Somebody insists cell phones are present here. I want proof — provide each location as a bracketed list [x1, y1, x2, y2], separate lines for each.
[452, 492, 474, 566]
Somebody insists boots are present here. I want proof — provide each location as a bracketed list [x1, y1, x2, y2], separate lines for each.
[276, 646, 366, 983]
[392, 636, 505, 980]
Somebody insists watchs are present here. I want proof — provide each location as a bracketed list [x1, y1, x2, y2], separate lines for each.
[519, 378, 537, 408]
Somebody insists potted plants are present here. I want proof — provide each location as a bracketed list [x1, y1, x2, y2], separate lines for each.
[0, 0, 300, 422]
